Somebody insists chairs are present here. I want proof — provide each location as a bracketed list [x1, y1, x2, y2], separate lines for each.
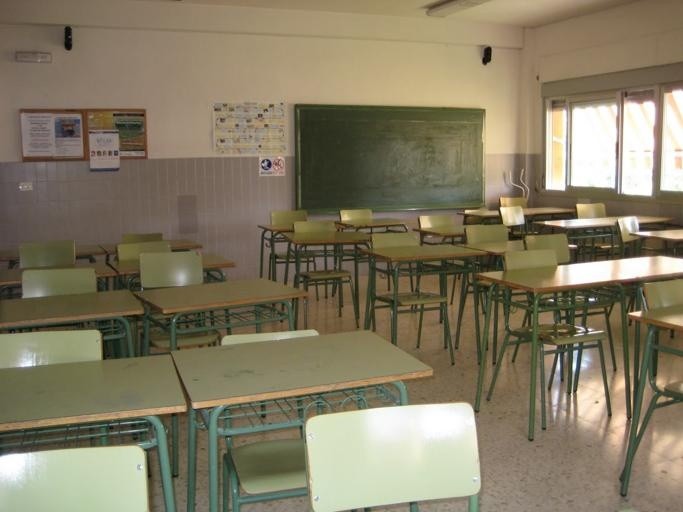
[0, 193, 682, 510]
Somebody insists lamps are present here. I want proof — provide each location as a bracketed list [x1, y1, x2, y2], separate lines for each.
[426, 0, 485, 17]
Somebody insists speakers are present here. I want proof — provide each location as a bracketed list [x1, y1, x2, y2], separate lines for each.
[63, 25, 72, 50]
[482, 46, 492, 64]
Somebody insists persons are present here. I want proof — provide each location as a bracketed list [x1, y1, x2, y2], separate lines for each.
[56, 118, 76, 137]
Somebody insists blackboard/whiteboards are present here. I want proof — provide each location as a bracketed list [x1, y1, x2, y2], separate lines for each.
[294, 104, 486, 216]
